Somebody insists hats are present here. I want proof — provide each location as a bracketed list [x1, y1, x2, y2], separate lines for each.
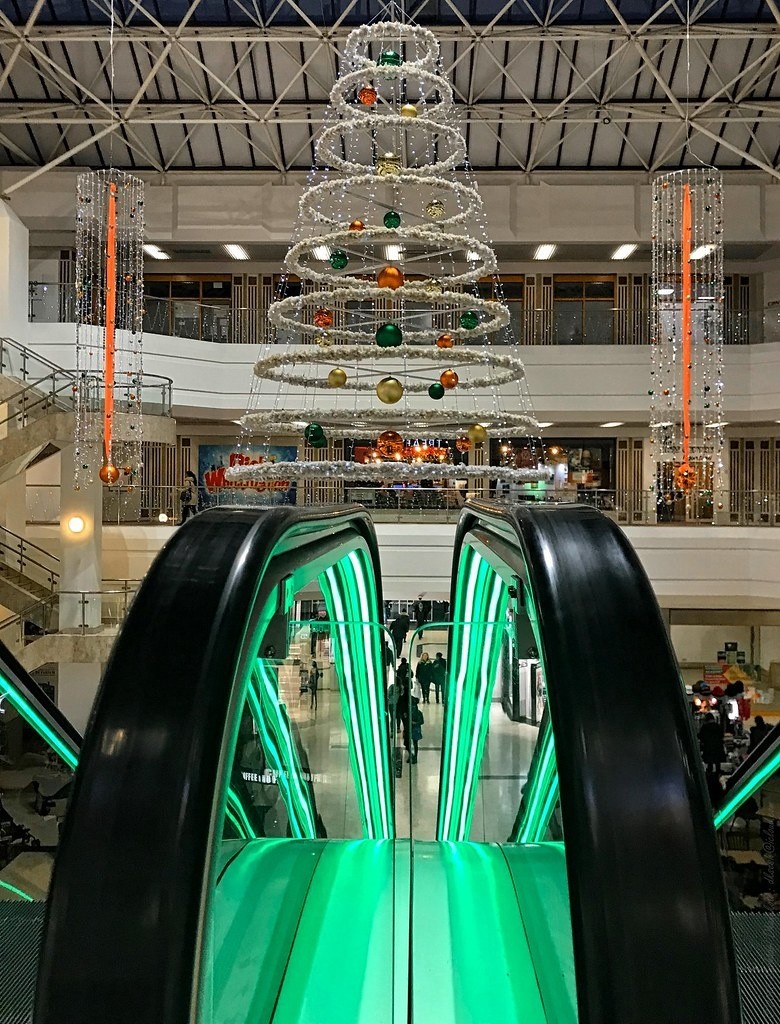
[692, 679, 744, 697]
[317, 605, 327, 611]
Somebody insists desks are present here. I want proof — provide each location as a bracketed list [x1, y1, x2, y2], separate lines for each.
[726, 850, 769, 880]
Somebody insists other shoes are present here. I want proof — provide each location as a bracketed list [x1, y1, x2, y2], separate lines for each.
[176, 517, 185, 526]
[422, 698, 426, 703]
[427, 699, 430, 705]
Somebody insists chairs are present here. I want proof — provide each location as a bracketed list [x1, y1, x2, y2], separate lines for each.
[730, 810, 760, 833]
[726, 831, 749, 851]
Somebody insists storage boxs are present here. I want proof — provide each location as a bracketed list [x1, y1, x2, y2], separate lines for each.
[717, 642, 745, 664]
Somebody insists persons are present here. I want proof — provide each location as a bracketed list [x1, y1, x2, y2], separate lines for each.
[697, 713, 726, 772]
[389, 616, 405, 659]
[177, 471, 198, 526]
[308, 661, 319, 710]
[384, 640, 447, 764]
[746, 716, 774, 755]
[414, 603, 424, 640]
[399, 608, 409, 643]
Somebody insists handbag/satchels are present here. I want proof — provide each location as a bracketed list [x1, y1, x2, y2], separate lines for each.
[180, 488, 192, 503]
[413, 678, 422, 700]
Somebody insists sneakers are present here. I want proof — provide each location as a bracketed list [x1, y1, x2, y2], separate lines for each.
[407, 755, 417, 764]
